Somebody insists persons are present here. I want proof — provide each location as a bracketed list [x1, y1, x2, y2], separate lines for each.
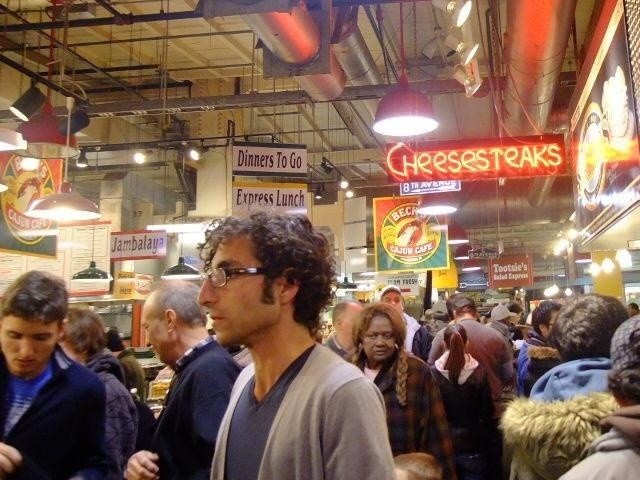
[122, 280, 241, 480]
[0, 269, 155, 479]
[323, 286, 640, 480]
[197, 212, 397, 480]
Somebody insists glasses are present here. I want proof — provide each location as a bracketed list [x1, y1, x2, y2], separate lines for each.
[198, 264, 269, 288]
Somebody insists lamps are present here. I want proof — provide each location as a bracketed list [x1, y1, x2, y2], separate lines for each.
[12, 0, 80, 159]
[372, 3, 482, 272]
[72, 222, 113, 281]
[161, 231, 201, 280]
[76, 133, 276, 168]
[314, 182, 325, 199]
[543, 228, 632, 297]
[0, 53, 89, 136]
[337, 261, 357, 291]
[321, 156, 353, 198]
[23, 97, 101, 222]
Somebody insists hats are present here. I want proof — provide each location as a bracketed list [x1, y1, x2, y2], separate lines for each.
[381, 285, 401, 296]
[105, 330, 125, 352]
[491, 305, 517, 321]
[432, 301, 449, 319]
[610, 315, 640, 369]
